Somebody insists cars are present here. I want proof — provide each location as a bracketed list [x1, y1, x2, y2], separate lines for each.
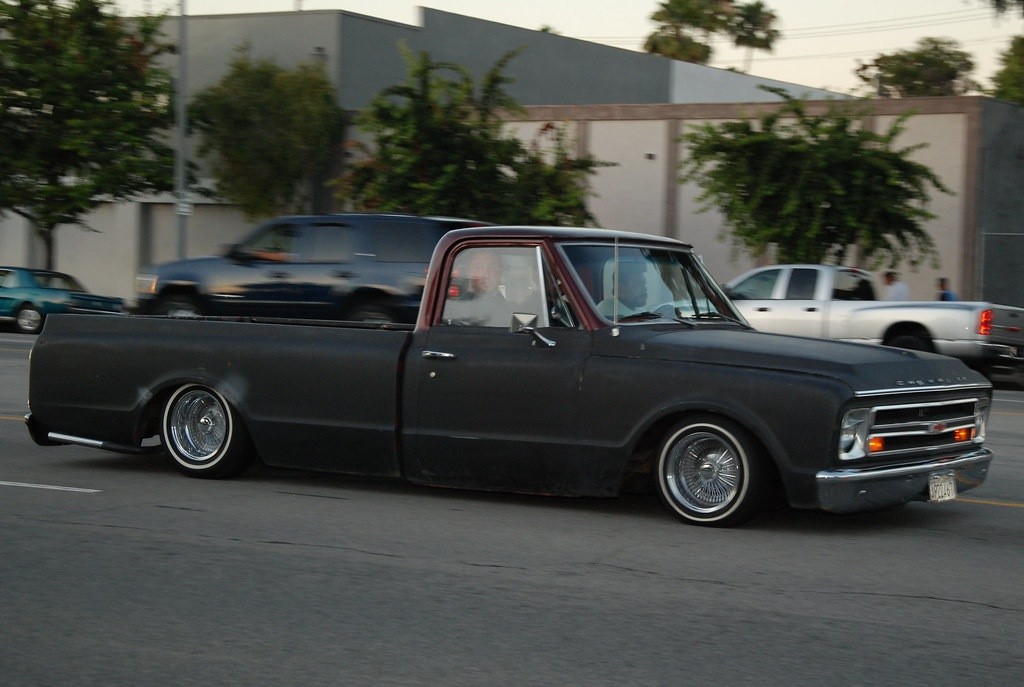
[0, 265, 130, 334]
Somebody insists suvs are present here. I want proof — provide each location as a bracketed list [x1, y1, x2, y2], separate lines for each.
[132, 210, 496, 326]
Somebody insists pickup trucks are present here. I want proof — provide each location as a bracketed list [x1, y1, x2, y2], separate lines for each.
[24, 226, 998, 530]
[651, 262, 1024, 369]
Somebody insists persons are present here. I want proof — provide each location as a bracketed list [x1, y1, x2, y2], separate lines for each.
[935, 277, 960, 301]
[249, 251, 301, 260]
[882, 271, 910, 301]
[453, 250, 681, 328]
